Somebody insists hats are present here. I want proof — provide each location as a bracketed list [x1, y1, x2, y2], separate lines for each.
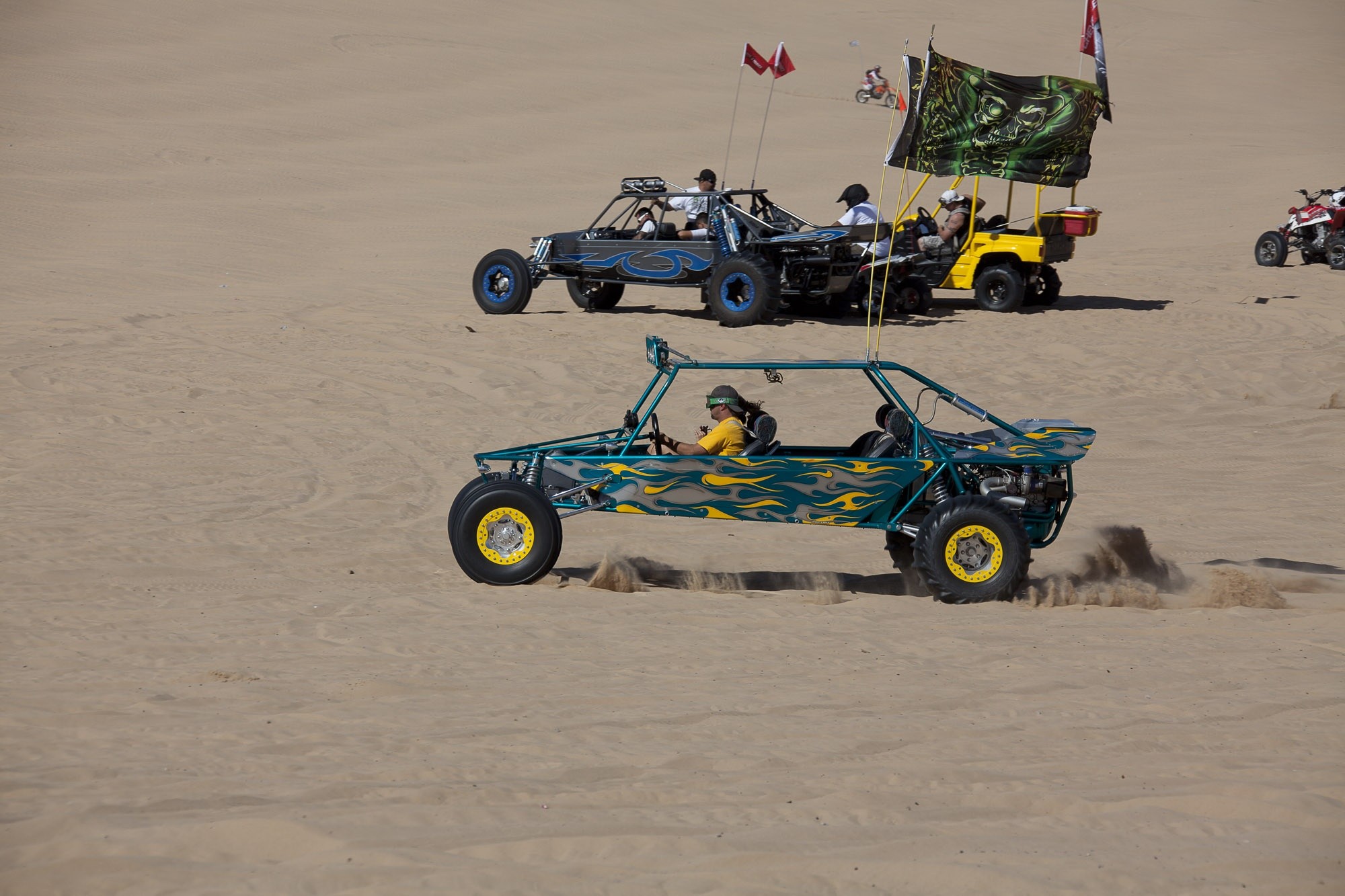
[694, 169, 716, 181]
[711, 385, 744, 412]
[941, 190, 965, 205]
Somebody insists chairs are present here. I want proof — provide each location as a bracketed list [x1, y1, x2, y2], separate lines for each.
[845, 404, 908, 458]
[641, 221, 676, 242]
[738, 411, 781, 457]
[959, 210, 1064, 247]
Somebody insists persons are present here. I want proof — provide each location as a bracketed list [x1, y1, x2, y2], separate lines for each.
[917, 191, 985, 261]
[831, 183, 890, 261]
[632, 207, 657, 239]
[651, 169, 720, 222]
[648, 384, 760, 456]
[678, 212, 715, 241]
[865, 65, 886, 89]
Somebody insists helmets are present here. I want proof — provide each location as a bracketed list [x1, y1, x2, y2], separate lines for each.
[836, 184, 869, 212]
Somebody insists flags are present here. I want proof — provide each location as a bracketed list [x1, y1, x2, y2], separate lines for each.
[908, 44, 1103, 179]
[744, 44, 769, 75]
[1080, 0, 1112, 124]
[768, 45, 795, 78]
[885, 54, 1076, 188]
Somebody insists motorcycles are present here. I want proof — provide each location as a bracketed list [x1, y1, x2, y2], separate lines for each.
[1255, 188, 1345, 271]
[855, 77, 899, 109]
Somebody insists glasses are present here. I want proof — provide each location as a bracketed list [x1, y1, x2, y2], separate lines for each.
[704, 180, 715, 183]
[637, 212, 649, 223]
[939, 199, 947, 207]
[706, 395, 712, 408]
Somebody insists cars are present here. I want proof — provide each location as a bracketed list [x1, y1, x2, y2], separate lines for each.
[446, 334, 1097, 604]
[889, 172, 1103, 309]
[471, 175, 894, 329]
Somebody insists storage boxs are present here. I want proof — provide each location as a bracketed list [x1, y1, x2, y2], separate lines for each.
[1064, 210, 1098, 236]
